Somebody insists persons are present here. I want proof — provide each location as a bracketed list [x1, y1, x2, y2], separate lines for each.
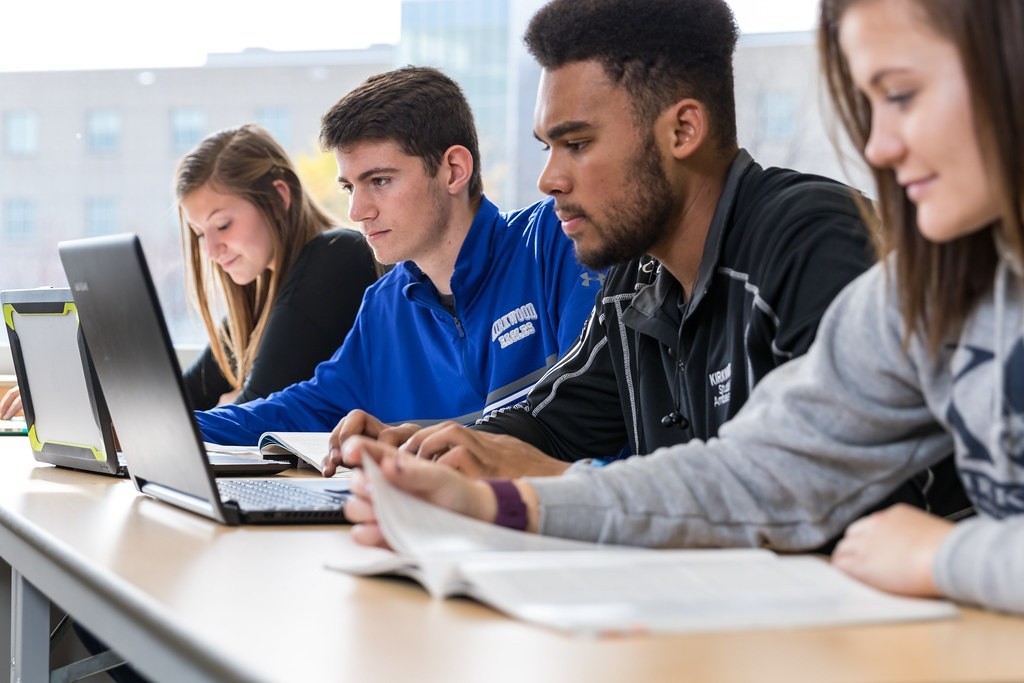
[321, 0, 952, 557]
[110, 66, 609, 451]
[0, 124, 385, 421]
[343, 1, 1024, 616]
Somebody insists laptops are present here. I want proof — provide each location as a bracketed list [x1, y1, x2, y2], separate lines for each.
[0, 287, 290, 479]
[0, 416, 29, 435]
[58, 234, 355, 526]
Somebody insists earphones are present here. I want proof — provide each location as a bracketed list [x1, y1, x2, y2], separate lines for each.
[661, 410, 677, 428]
[677, 413, 689, 431]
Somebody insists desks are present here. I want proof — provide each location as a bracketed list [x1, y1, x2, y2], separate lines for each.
[0, 420, 1024, 683]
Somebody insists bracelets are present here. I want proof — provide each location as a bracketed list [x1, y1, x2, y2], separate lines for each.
[479, 479, 528, 531]
